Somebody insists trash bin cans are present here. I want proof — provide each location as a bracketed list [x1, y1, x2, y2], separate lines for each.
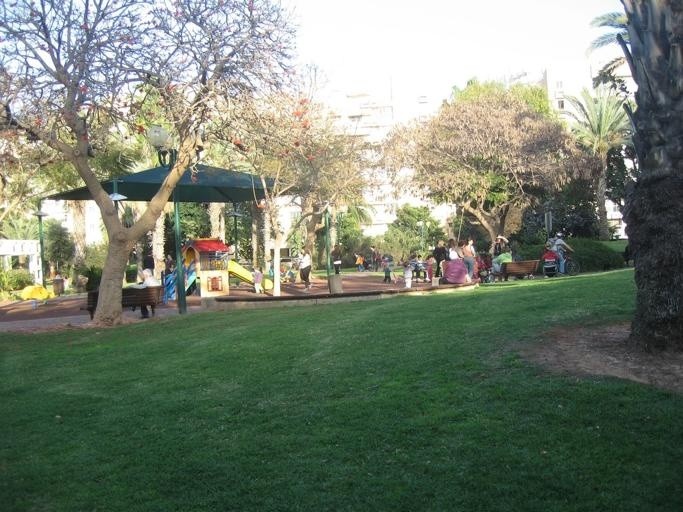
[52, 278, 64, 296]
[328, 273, 344, 294]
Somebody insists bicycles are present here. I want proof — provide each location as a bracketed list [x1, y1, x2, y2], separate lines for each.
[539, 248, 582, 276]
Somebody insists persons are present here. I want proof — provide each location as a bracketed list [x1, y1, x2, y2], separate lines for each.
[332, 244, 341, 273]
[63, 276, 69, 288]
[299, 246, 312, 289]
[55, 271, 61, 279]
[131, 249, 174, 318]
[269, 260, 273, 276]
[253, 268, 264, 293]
[354, 232, 574, 288]
[280, 265, 285, 276]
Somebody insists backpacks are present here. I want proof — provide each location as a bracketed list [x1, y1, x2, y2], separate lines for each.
[545, 237, 558, 251]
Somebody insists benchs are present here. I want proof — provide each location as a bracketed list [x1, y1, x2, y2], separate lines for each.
[488, 259, 541, 281]
[80, 287, 161, 319]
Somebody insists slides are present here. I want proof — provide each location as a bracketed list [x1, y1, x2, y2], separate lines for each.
[229, 260, 275, 289]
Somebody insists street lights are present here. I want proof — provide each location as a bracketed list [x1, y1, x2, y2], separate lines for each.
[322, 203, 348, 273]
[146, 122, 189, 316]
[416, 221, 431, 254]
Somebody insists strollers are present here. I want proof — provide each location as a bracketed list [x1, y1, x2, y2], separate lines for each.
[476, 252, 495, 283]
